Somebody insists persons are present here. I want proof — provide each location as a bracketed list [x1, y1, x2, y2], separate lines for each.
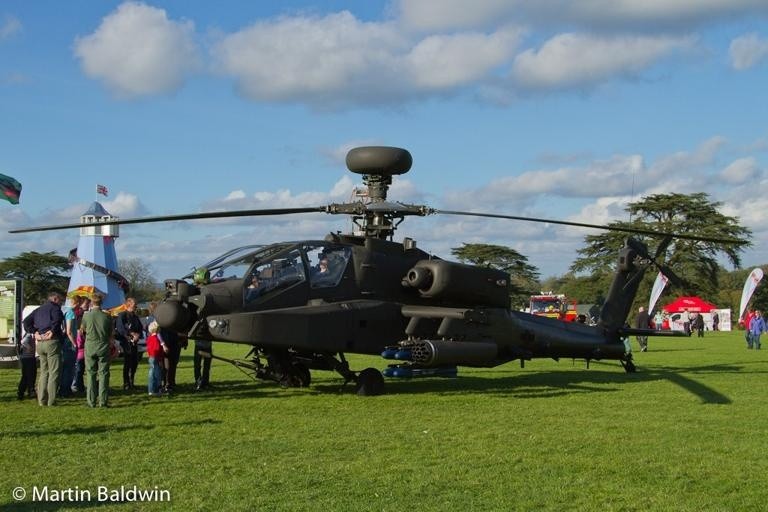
[681, 308, 692, 333]
[634, 306, 650, 353]
[748, 310, 767, 350]
[653, 310, 663, 332]
[711, 311, 720, 331]
[624, 320, 633, 353]
[694, 311, 704, 338]
[13, 284, 214, 408]
[247, 271, 261, 288]
[316, 258, 329, 274]
[737, 306, 763, 348]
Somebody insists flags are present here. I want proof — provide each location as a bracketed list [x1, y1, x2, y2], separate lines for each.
[96, 184, 109, 198]
[0, 175, 22, 205]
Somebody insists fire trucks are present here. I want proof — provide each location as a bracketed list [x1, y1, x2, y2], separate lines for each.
[526, 290, 578, 324]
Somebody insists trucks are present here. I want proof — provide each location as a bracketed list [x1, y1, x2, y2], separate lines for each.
[18, 304, 146, 360]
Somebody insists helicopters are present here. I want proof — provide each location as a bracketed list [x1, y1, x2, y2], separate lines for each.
[9, 144, 750, 393]
